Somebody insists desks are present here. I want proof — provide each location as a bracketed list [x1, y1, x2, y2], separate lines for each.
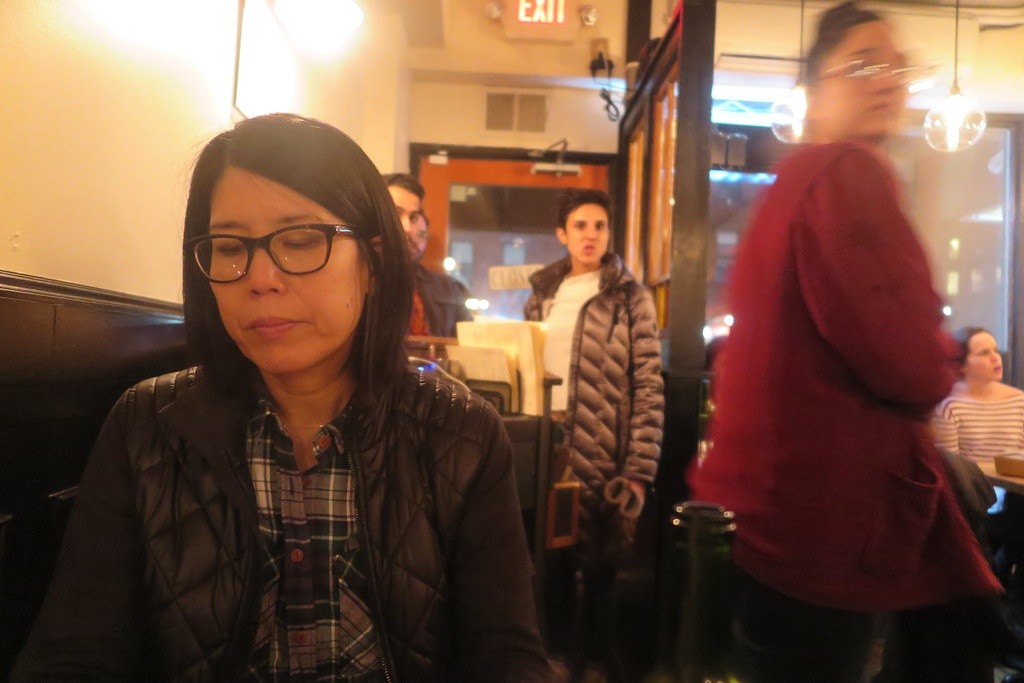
[977, 460, 1024, 592]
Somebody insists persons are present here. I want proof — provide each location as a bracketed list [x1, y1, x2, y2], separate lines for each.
[930, 326, 1024, 577]
[523, 189, 665, 683]
[7, 114, 554, 683]
[690, 0, 1005, 683]
[383, 173, 474, 360]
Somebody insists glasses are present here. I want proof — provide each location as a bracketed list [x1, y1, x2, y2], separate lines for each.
[186, 222, 361, 285]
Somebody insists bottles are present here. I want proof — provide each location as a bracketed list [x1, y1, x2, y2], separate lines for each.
[630, 501, 748, 683]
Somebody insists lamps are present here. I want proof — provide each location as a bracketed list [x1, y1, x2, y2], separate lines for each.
[484, 0, 598, 46]
[769, 0, 807, 145]
[923, 0, 987, 153]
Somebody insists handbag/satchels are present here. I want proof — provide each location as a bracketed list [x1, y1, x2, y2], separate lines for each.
[937, 444, 998, 518]
